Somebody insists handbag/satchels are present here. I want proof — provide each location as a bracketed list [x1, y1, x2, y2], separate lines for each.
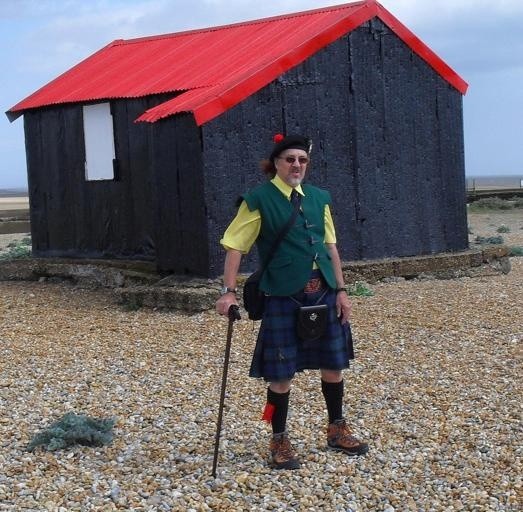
[243, 271, 268, 319]
[293, 292, 330, 343]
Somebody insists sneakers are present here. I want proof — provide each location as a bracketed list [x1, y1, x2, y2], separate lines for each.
[327, 418, 369, 455]
[271, 433, 300, 469]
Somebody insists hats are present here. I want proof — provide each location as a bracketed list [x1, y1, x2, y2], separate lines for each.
[268, 134, 312, 161]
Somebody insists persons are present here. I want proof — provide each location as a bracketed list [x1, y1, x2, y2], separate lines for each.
[216, 132, 368, 470]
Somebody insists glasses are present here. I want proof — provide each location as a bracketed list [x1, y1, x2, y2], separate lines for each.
[277, 156, 308, 163]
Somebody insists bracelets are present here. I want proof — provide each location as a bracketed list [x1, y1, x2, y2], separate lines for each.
[222, 287, 237, 293]
[335, 287, 347, 295]
